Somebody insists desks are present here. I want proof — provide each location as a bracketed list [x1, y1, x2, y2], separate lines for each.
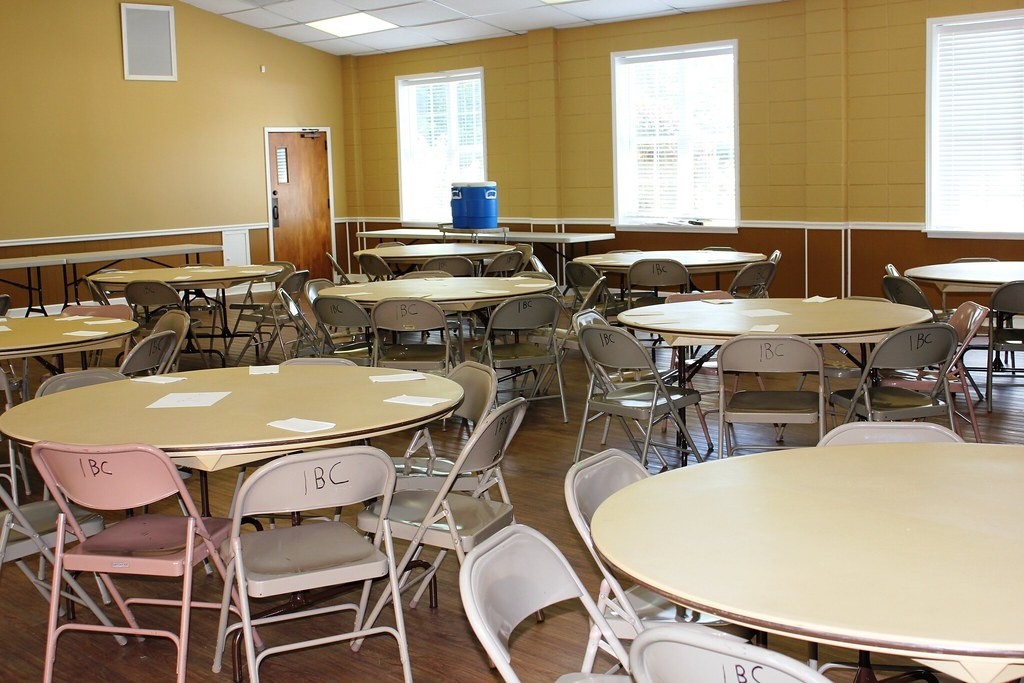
[618, 299, 933, 468]
[572, 250, 767, 363]
[904, 261, 1024, 398]
[356, 228, 615, 298]
[36, 242, 223, 312]
[0, 315, 140, 403]
[87, 266, 282, 369]
[0, 257, 67, 317]
[353, 243, 517, 346]
[318, 277, 557, 381]
[0, 365, 466, 683]
[589, 441, 1024, 683]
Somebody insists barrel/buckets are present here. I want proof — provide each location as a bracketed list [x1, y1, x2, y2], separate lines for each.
[451, 181, 498, 229]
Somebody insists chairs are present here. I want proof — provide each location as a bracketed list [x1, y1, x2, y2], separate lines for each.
[0, 243, 1024, 683]
